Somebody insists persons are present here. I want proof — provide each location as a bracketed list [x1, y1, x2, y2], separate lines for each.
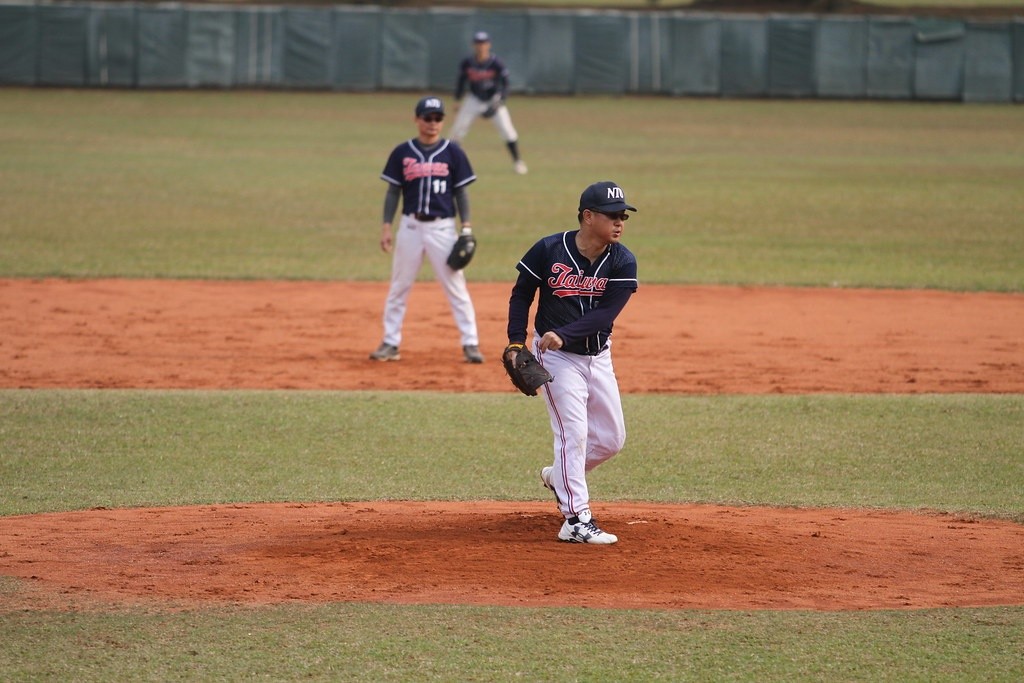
[449, 31, 528, 175]
[503, 181, 638, 544]
[369, 96, 484, 363]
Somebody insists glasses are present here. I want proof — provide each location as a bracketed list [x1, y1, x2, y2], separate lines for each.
[590, 210, 629, 220]
[422, 115, 442, 122]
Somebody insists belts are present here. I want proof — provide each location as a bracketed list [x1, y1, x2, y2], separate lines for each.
[402, 209, 447, 221]
[578, 345, 609, 356]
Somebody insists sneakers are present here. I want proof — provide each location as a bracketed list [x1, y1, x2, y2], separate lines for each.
[539, 466, 566, 518]
[368, 342, 401, 362]
[557, 509, 618, 545]
[462, 345, 482, 364]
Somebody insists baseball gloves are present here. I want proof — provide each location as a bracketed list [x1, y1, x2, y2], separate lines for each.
[446, 235, 477, 272]
[482, 105, 497, 118]
[502, 342, 555, 397]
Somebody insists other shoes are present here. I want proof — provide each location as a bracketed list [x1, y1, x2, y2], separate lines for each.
[510, 159, 528, 176]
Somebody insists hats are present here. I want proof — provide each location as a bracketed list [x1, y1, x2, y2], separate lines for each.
[578, 181, 637, 214]
[472, 31, 490, 43]
[416, 97, 444, 116]
